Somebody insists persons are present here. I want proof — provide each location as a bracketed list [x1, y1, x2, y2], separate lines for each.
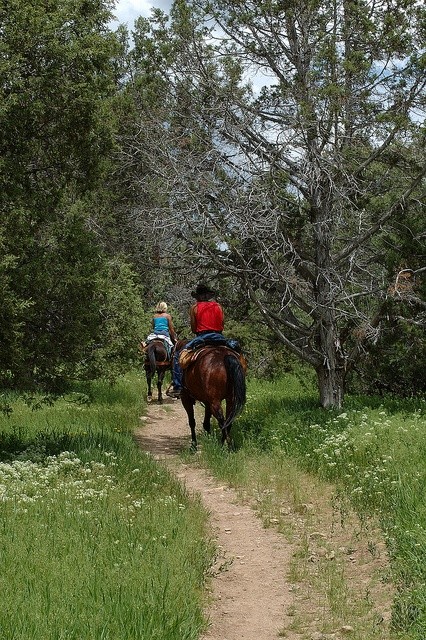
[166, 282, 238, 398]
[152, 302, 180, 345]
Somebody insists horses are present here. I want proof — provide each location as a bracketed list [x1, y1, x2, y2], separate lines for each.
[175, 336, 248, 455]
[142, 330, 179, 404]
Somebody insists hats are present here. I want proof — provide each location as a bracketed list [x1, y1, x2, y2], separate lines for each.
[191, 284, 215, 301]
